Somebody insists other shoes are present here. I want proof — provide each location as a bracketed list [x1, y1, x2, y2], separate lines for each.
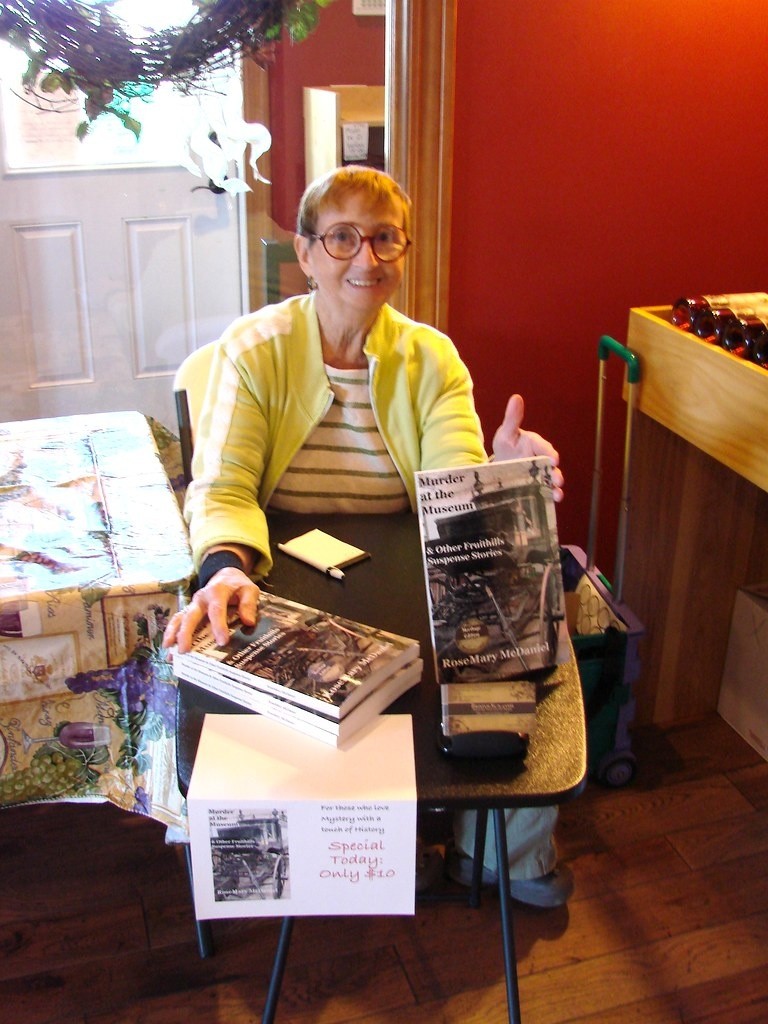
[444, 840, 574, 907]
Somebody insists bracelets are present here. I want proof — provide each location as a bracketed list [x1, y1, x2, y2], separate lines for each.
[198, 549, 245, 588]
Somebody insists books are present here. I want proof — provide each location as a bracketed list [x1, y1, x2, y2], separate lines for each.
[168, 590, 424, 747]
[415, 455, 572, 685]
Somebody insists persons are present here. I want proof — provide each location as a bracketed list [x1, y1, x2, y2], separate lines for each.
[162, 164, 576, 907]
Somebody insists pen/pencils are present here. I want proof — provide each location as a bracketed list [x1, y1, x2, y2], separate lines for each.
[277, 542, 345, 581]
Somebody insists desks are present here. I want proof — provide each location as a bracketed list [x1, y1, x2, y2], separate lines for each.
[619, 292, 768, 761]
[0, 412, 214, 959]
[177, 514, 586, 1024]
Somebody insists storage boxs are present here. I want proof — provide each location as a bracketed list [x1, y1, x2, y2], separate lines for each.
[717, 582, 768, 761]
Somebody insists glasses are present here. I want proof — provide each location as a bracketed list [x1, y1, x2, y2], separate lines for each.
[310, 224, 413, 263]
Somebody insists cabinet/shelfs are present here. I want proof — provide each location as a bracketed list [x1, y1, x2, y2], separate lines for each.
[303, 85, 384, 192]
